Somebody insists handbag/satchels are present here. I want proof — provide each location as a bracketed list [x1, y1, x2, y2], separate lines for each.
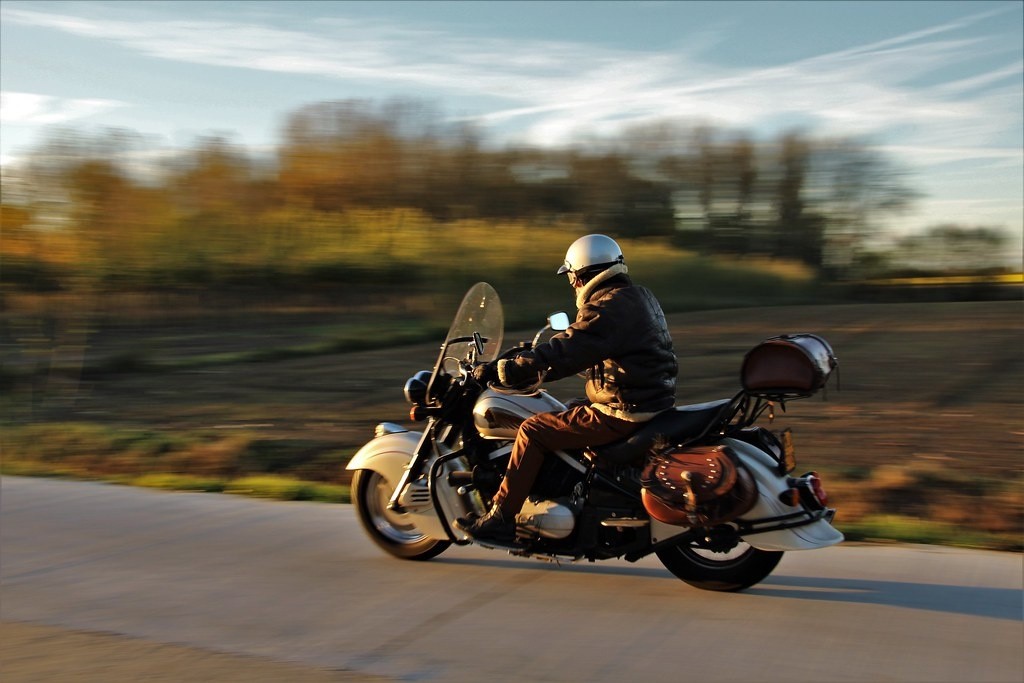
[644, 447, 756, 527]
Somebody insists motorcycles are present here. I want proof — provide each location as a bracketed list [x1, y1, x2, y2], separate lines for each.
[345, 280, 846, 592]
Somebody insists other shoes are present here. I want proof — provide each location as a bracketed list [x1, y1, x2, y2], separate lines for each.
[452, 505, 515, 541]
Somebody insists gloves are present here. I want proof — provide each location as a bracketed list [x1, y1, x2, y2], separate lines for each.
[473, 363, 499, 381]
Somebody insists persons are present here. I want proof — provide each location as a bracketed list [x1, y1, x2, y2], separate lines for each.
[452, 234, 681, 548]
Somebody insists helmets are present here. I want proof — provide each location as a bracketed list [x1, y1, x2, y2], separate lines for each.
[557, 234, 622, 275]
